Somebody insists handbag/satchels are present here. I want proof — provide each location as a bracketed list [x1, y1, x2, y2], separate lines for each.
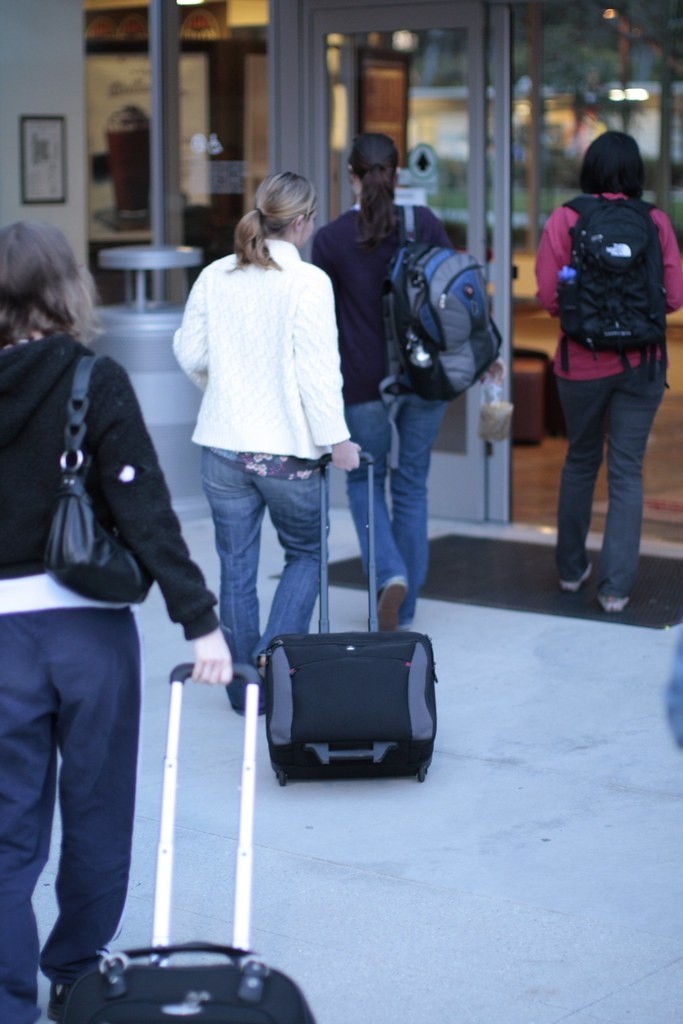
[42, 351, 160, 606]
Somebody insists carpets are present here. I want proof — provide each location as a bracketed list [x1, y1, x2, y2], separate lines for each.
[267, 531, 683, 631]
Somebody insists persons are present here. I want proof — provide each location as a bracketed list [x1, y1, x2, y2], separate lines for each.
[312, 132, 507, 631]
[172, 171, 359, 717]
[535, 130, 683, 611]
[0, 222, 232, 1024]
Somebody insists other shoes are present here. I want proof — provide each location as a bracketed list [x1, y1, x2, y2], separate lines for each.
[596, 590, 630, 614]
[558, 561, 594, 592]
[45, 981, 71, 1022]
[366, 575, 408, 632]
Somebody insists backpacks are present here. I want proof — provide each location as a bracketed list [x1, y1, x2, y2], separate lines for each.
[556, 193, 678, 389]
[353, 205, 504, 468]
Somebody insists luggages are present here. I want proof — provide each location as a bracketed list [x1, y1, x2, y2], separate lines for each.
[70, 664, 315, 1024]
[256, 450, 438, 781]
